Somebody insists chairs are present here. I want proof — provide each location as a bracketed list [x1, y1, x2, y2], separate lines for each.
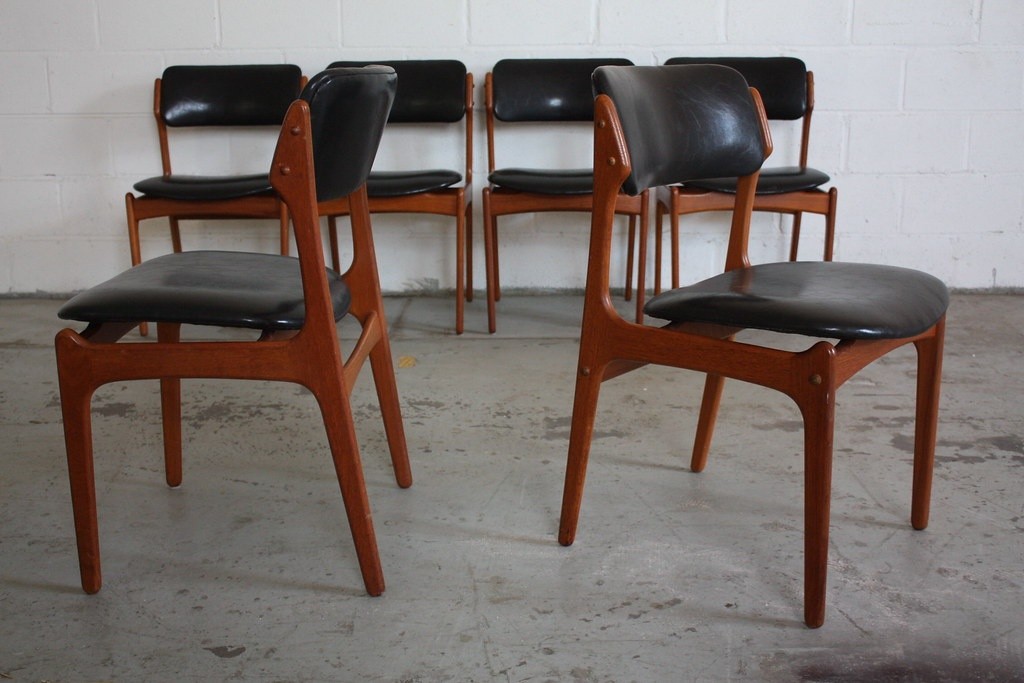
[54, 66, 411, 600]
[556, 63, 951, 631]
[483, 58, 650, 333]
[125, 64, 308, 337]
[325, 60, 476, 334]
[655, 57, 838, 297]
